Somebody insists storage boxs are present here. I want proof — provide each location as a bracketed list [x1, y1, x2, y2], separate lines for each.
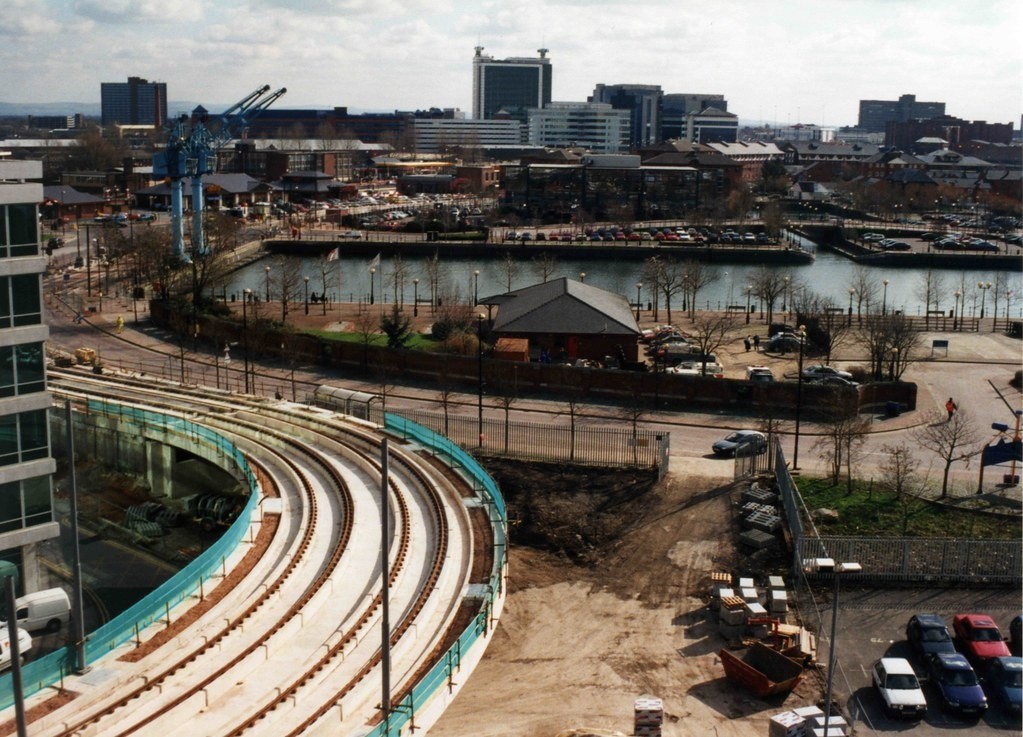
[767, 706, 846, 737]
[634, 697, 664, 737]
[815, 558, 864, 574]
[712, 573, 790, 639]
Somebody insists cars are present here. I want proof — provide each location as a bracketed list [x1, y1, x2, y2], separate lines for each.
[804, 364, 861, 387]
[862, 213, 1023, 251]
[273, 190, 506, 232]
[984, 654, 1022, 724]
[505, 227, 769, 242]
[952, 613, 1013, 667]
[765, 321, 809, 353]
[639, 324, 725, 379]
[905, 613, 957, 670]
[1008, 615, 1023, 657]
[928, 652, 988, 722]
[93, 210, 156, 223]
[872, 657, 928, 722]
[712, 430, 768, 459]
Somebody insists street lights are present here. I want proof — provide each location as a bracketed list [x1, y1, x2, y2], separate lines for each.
[636, 282, 643, 322]
[475, 313, 486, 447]
[847, 287, 856, 328]
[782, 276, 788, 312]
[304, 276, 310, 315]
[474, 270, 480, 307]
[882, 279, 889, 316]
[978, 280, 992, 319]
[243, 287, 251, 393]
[953, 291, 961, 331]
[413, 279, 419, 317]
[683, 274, 689, 312]
[265, 267, 271, 303]
[745, 285, 751, 326]
[370, 268, 376, 305]
[580, 272, 586, 282]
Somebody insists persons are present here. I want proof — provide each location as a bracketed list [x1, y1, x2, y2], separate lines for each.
[75, 311, 84, 324]
[753, 333, 760, 352]
[746, 335, 752, 352]
[945, 397, 957, 421]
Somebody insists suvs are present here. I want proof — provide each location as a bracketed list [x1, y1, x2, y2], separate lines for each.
[0, 626, 33, 672]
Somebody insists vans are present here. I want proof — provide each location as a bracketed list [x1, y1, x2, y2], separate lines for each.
[745, 364, 773, 380]
[0, 586, 73, 634]
